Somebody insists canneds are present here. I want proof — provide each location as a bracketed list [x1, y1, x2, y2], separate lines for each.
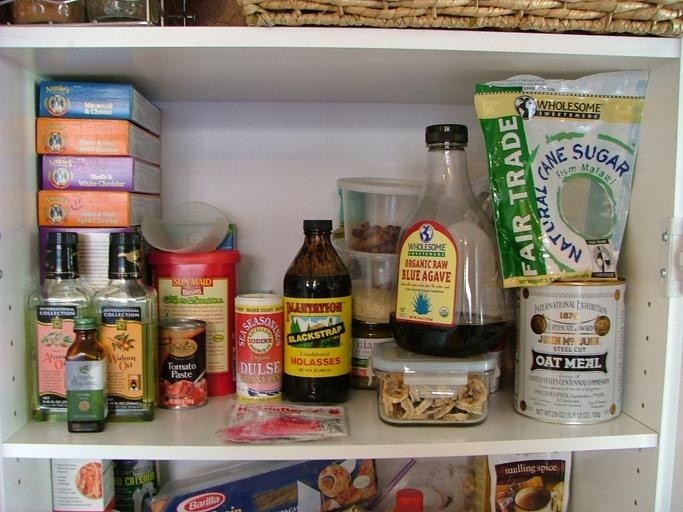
[512, 277, 627, 425]
[349, 322, 392, 390]
[160, 318, 207, 410]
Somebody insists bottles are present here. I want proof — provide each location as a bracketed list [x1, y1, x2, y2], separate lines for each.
[389, 122, 514, 359]
[23, 231, 93, 424]
[282, 219, 353, 404]
[64, 316, 109, 434]
[92, 231, 157, 422]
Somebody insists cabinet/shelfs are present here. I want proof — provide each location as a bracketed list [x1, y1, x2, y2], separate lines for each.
[0, 22, 683, 512]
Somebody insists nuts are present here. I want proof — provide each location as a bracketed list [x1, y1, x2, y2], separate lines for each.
[382, 374, 487, 421]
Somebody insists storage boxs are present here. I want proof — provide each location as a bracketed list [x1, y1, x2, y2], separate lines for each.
[331, 177, 503, 428]
[34, 80, 163, 294]
